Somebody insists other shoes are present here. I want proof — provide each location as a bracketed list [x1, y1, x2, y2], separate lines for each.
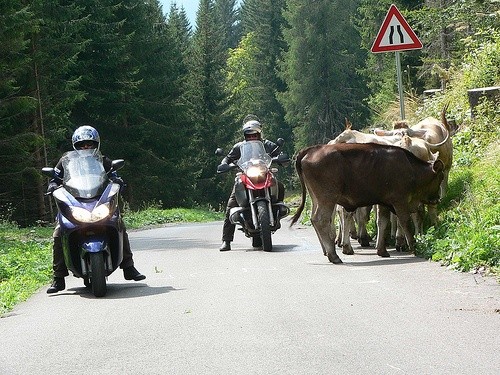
[124, 267, 146, 281]
[279, 202, 287, 215]
[219, 241, 231, 251]
[47, 278, 65, 294]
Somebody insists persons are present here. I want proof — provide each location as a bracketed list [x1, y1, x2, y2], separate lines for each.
[46, 125, 146, 294]
[217, 120, 287, 251]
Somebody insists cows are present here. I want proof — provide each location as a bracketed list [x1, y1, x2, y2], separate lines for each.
[287, 142, 444, 264]
[327, 101, 453, 258]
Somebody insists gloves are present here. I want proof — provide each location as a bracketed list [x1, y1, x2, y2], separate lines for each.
[47, 183, 57, 196]
[270, 147, 280, 157]
[113, 176, 124, 184]
[218, 163, 228, 170]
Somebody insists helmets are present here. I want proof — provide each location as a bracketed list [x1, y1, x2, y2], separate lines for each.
[71, 125, 101, 157]
[243, 120, 262, 135]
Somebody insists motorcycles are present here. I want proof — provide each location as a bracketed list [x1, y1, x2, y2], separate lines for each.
[41, 148, 126, 296]
[214, 137, 286, 252]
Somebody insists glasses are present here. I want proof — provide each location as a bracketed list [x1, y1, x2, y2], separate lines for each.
[79, 143, 93, 147]
